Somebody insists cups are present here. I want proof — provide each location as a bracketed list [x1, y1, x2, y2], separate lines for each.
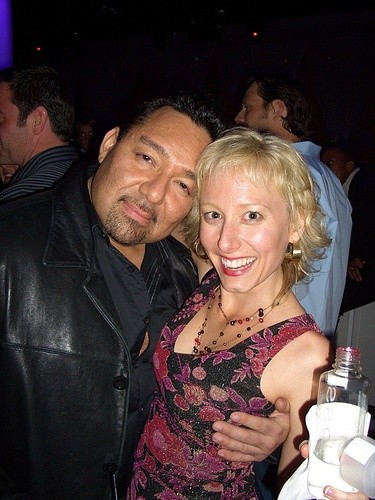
[305, 402, 371, 500]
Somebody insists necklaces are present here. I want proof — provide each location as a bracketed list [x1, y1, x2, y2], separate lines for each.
[193, 283, 273, 355]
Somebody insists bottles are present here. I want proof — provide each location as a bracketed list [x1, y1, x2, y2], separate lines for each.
[312, 347, 371, 466]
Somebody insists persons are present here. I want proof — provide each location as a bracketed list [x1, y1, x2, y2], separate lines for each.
[0, 84, 291, 500]
[75, 118, 99, 155]
[235, 73, 354, 337]
[0, 165, 19, 186]
[124, 128, 369, 500]
[0, 63, 80, 201]
[321, 142, 375, 415]
